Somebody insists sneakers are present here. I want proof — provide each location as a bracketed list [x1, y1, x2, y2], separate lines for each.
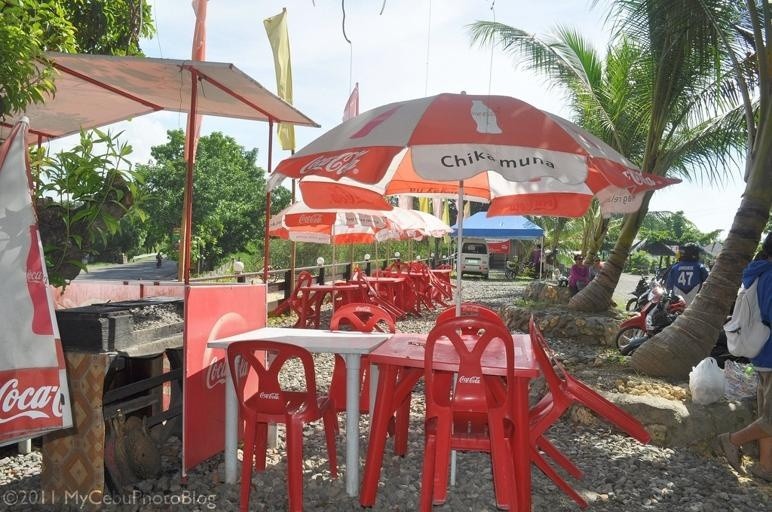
[719, 432, 746, 475]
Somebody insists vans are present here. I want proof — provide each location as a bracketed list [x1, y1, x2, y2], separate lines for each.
[461, 238, 492, 279]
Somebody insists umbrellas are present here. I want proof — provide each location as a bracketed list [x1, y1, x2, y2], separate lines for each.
[264, 91, 684, 487]
[0, 115, 74, 448]
[269, 206, 454, 321]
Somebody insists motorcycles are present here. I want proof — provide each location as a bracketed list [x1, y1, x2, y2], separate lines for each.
[615, 273, 686, 357]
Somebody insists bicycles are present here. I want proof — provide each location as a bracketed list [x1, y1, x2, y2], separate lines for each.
[505, 265, 520, 280]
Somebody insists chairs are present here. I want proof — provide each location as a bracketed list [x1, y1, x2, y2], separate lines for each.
[221, 256, 654, 509]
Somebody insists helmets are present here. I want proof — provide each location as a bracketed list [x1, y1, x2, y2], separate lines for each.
[679, 242, 705, 263]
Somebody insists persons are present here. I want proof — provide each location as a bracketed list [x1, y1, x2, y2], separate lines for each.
[156, 252, 163, 265]
[586, 254, 603, 281]
[568, 254, 590, 291]
[710, 231, 772, 485]
[532, 240, 545, 281]
[665, 241, 710, 306]
